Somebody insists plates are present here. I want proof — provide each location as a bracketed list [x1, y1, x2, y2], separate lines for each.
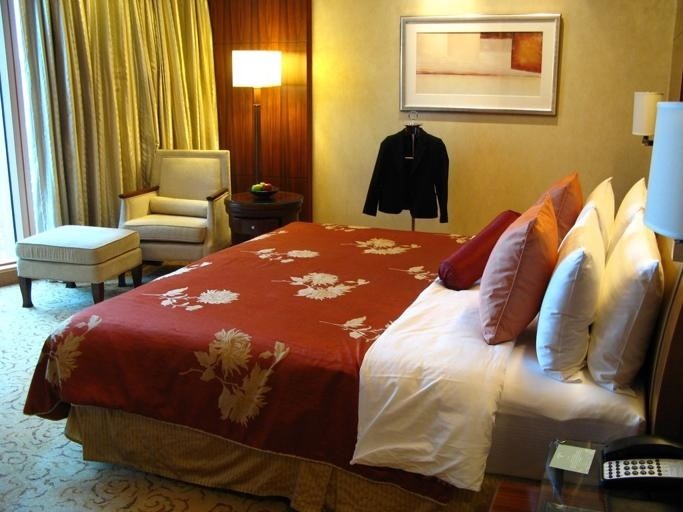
[253, 198, 275, 203]
[249, 187, 279, 196]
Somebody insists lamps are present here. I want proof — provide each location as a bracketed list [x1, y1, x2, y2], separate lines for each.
[231, 49, 282, 184]
[632, 88, 683, 247]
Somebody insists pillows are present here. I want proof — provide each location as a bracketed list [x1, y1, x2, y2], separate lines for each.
[587, 207, 665, 391]
[439, 209, 522, 291]
[539, 173, 585, 249]
[479, 190, 560, 345]
[606, 176, 648, 263]
[535, 206, 606, 382]
[585, 177, 616, 256]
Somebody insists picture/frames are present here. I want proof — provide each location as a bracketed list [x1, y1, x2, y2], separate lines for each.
[400, 13, 561, 115]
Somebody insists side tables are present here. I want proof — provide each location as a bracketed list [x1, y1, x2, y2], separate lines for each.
[224, 191, 303, 245]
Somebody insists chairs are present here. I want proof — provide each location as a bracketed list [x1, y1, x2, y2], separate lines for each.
[117, 149, 233, 261]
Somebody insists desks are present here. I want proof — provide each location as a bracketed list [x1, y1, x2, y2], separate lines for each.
[536, 437, 682, 512]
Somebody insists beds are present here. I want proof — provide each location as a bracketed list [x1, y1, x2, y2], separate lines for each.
[58, 220, 682, 511]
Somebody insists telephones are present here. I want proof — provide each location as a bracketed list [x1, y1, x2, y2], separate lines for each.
[599, 434, 683, 492]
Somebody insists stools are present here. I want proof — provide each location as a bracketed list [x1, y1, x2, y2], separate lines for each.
[15, 224, 144, 307]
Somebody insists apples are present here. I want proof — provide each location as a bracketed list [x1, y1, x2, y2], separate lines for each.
[251, 181, 273, 191]
